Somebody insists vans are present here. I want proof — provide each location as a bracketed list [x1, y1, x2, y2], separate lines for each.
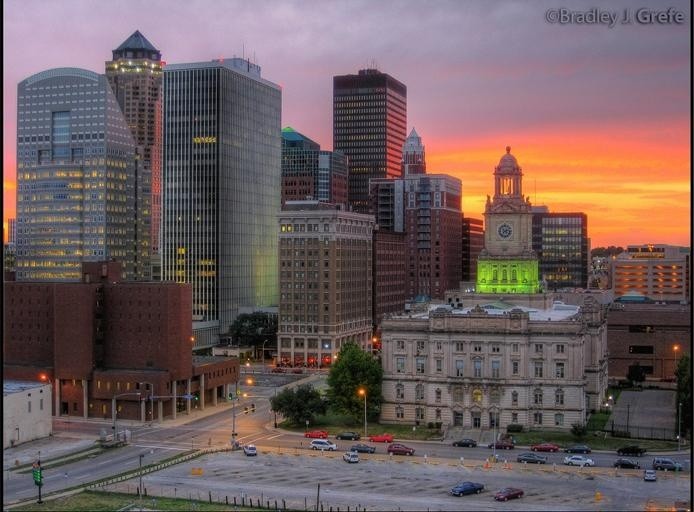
[343, 452, 358, 463]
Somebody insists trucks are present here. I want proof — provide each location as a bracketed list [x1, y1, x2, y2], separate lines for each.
[101, 430, 131, 449]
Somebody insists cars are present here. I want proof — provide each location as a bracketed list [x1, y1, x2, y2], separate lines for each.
[305, 429, 328, 438]
[645, 468, 657, 480]
[370, 432, 393, 443]
[495, 487, 524, 502]
[292, 366, 306, 374]
[453, 438, 596, 467]
[449, 480, 485, 497]
[351, 443, 376, 453]
[272, 366, 287, 373]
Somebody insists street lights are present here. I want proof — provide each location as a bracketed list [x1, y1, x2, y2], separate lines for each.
[262, 339, 269, 372]
[608, 394, 616, 436]
[370, 337, 377, 355]
[359, 386, 368, 441]
[140, 381, 154, 424]
[138, 453, 145, 499]
[40, 375, 52, 384]
[235, 378, 252, 404]
[672, 345, 680, 372]
[492, 383, 499, 465]
[679, 402, 683, 453]
[111, 391, 141, 428]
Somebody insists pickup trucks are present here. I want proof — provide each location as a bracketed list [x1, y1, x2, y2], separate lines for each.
[617, 445, 646, 457]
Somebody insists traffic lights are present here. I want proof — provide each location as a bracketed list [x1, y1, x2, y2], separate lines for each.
[195, 392, 200, 401]
[228, 393, 232, 400]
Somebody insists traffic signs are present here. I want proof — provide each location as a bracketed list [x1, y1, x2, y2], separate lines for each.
[32, 465, 41, 482]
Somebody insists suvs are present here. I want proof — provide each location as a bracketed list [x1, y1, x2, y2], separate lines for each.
[243, 444, 257, 456]
[615, 459, 641, 469]
[654, 457, 683, 471]
[336, 431, 360, 441]
[309, 439, 337, 452]
[387, 443, 415, 456]
